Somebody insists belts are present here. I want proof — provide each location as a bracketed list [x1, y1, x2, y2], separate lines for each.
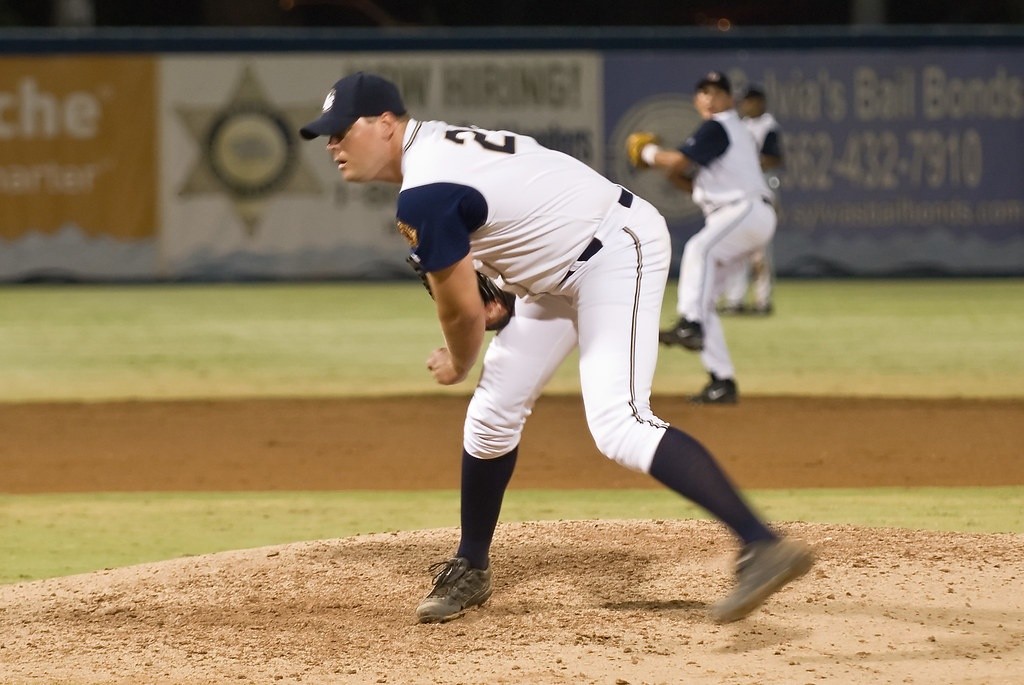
[763, 197, 771, 203]
[558, 189, 633, 287]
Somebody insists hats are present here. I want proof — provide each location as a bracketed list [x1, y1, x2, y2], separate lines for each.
[695, 70, 730, 90]
[299, 71, 405, 141]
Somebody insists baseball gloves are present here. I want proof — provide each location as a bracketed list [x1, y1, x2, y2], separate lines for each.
[406, 256, 515, 331]
[625, 131, 663, 169]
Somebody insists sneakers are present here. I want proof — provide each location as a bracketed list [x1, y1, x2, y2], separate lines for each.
[659, 317, 704, 350]
[416, 556, 493, 623]
[710, 536, 814, 621]
[692, 373, 737, 404]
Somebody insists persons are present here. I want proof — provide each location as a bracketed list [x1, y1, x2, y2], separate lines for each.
[716, 86, 784, 316]
[623, 71, 778, 405]
[299, 70, 815, 625]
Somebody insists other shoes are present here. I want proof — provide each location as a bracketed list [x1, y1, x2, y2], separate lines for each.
[750, 303, 772, 313]
[716, 303, 743, 314]
